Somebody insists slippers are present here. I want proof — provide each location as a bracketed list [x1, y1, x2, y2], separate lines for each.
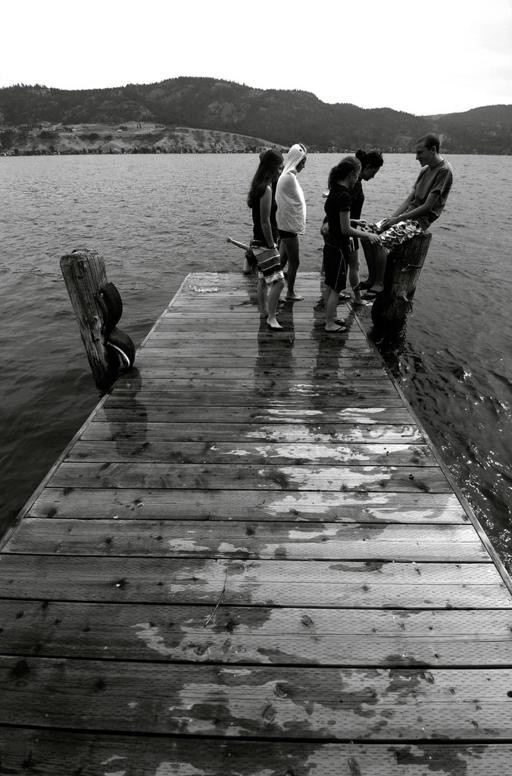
[352, 281, 372, 291]
[324, 316, 346, 325]
[324, 324, 347, 333]
[360, 288, 385, 300]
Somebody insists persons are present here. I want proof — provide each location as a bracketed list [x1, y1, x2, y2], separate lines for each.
[337, 150, 384, 308]
[351, 133, 454, 302]
[273, 141, 310, 302]
[320, 154, 383, 334]
[246, 147, 285, 331]
